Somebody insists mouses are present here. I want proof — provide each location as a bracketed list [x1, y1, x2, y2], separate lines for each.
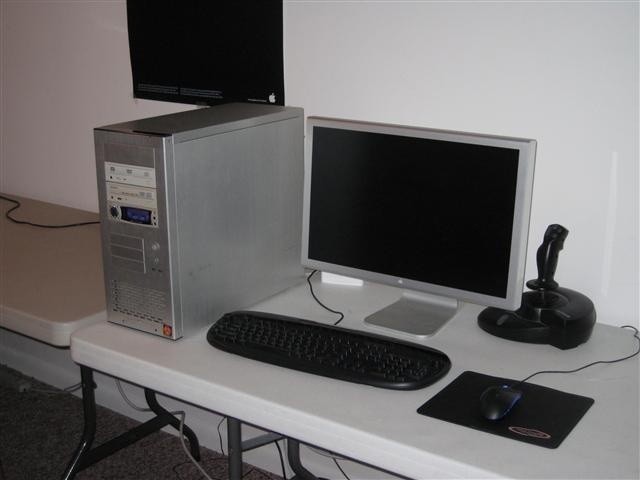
[479, 385, 524, 420]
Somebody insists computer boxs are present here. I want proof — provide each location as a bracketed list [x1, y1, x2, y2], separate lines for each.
[93, 102, 305, 341]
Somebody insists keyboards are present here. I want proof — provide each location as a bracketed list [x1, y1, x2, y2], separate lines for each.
[206, 311, 452, 391]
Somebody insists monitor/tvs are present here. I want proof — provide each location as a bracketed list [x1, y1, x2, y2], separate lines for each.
[299, 115, 538, 340]
